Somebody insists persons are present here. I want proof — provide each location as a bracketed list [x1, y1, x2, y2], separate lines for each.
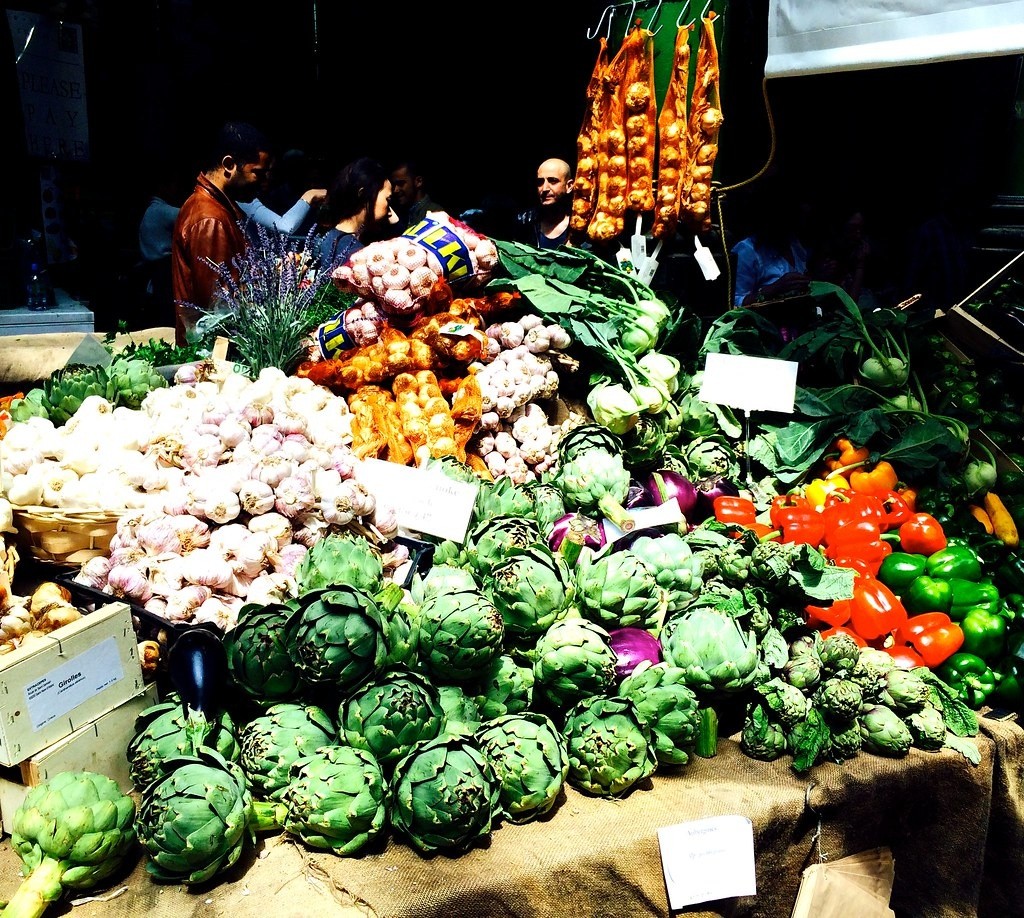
[516, 158, 574, 250]
[730, 207, 812, 307]
[312, 158, 392, 272]
[235, 188, 327, 248]
[818, 208, 882, 314]
[172, 120, 272, 350]
[390, 159, 446, 229]
[138, 196, 181, 295]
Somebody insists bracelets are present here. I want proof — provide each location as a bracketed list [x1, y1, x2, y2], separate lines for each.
[300, 198, 311, 206]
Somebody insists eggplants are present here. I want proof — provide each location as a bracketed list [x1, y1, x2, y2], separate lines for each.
[623, 470, 740, 534]
[546, 507, 606, 554]
[605, 627, 663, 683]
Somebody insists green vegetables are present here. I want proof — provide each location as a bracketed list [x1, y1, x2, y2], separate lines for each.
[488, 239, 1001, 500]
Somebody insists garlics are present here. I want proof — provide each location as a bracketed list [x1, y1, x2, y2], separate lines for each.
[0, 211, 603, 685]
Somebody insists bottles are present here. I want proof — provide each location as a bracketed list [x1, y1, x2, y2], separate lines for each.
[24, 263, 50, 311]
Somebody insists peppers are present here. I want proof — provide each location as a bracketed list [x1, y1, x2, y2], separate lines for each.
[710, 338, 1024, 720]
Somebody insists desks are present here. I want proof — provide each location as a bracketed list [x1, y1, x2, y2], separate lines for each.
[1, 705, 1023, 917]
[0, 285, 95, 336]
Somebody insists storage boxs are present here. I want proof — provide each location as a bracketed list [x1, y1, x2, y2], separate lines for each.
[54, 536, 437, 679]
[16, 551, 80, 585]
[969, 428, 1024, 483]
[947, 250, 1024, 368]
[0, 602, 161, 796]
